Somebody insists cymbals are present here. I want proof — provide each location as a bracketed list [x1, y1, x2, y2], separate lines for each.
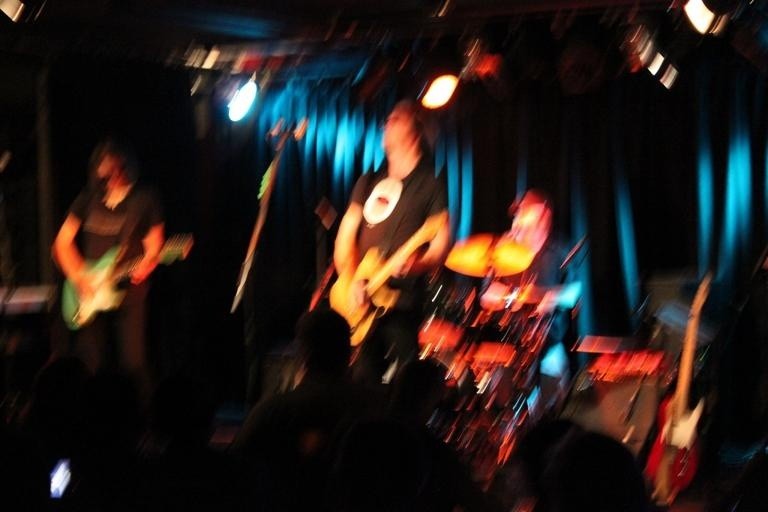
[446, 236, 534, 277]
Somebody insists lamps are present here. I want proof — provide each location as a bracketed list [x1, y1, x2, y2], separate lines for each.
[666, 0, 751, 37]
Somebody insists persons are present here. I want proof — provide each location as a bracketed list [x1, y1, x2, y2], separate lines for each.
[325, 96, 451, 386]
[51, 143, 165, 402]
[468, 188, 584, 421]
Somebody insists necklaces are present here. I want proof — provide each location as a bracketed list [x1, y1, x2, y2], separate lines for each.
[0, 254, 768, 511]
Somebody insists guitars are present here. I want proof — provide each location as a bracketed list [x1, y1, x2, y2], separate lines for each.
[61, 235, 195, 332]
[329, 210, 450, 346]
[647, 277, 710, 504]
